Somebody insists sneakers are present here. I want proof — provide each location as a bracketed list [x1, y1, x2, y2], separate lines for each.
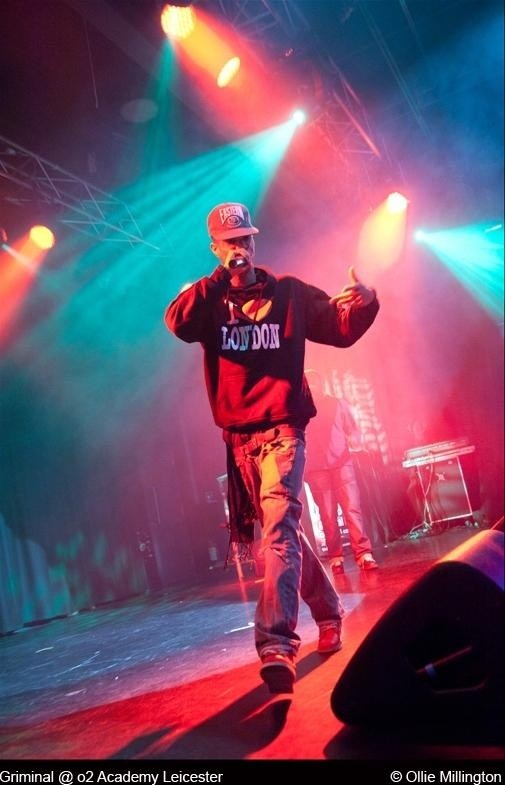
[357, 553, 379, 571]
[316, 623, 343, 654]
[331, 561, 345, 576]
[258, 656, 299, 695]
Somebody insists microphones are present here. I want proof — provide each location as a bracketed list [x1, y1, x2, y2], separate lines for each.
[229, 257, 248, 269]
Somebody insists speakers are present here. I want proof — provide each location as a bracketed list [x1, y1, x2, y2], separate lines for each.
[330, 529, 505, 730]
[490, 516, 505, 533]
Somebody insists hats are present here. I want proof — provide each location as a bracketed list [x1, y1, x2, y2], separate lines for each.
[206, 201, 259, 241]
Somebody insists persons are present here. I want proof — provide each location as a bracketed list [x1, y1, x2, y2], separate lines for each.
[162, 201, 381, 694]
[301, 370, 380, 571]
